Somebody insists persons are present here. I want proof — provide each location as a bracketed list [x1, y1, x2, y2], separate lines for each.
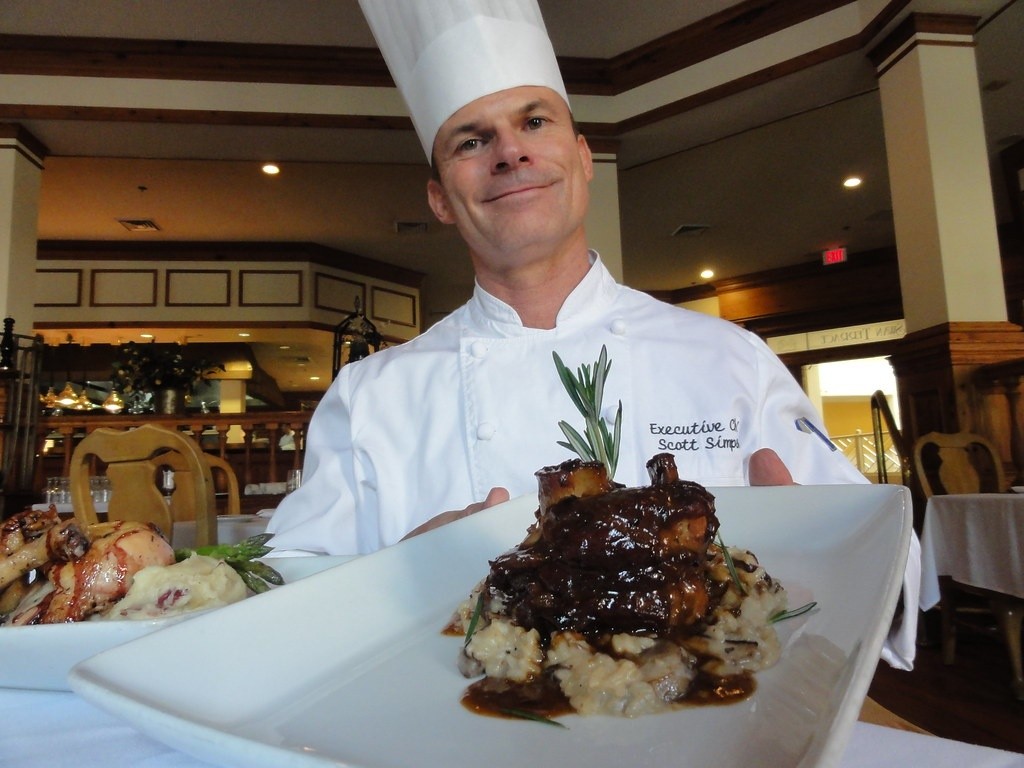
[251, 1, 922, 676]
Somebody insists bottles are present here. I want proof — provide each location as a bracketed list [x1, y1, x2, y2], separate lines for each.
[160, 469, 176, 495]
[46, 475, 111, 504]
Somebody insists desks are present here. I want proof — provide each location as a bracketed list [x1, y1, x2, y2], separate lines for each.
[174, 513, 270, 551]
[921, 493, 1024, 709]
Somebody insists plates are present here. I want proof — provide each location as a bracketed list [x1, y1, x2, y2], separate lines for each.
[216, 515, 253, 522]
[0, 554, 374, 691]
[1011, 487, 1024, 493]
[71, 484, 915, 767]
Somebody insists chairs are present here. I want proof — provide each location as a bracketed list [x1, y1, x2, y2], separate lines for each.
[69, 423, 240, 559]
[912, 432, 1005, 501]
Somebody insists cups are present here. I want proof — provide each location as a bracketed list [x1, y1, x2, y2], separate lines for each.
[286, 470, 302, 497]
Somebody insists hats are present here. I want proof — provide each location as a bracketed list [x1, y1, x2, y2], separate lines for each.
[358, 0, 573, 168]
